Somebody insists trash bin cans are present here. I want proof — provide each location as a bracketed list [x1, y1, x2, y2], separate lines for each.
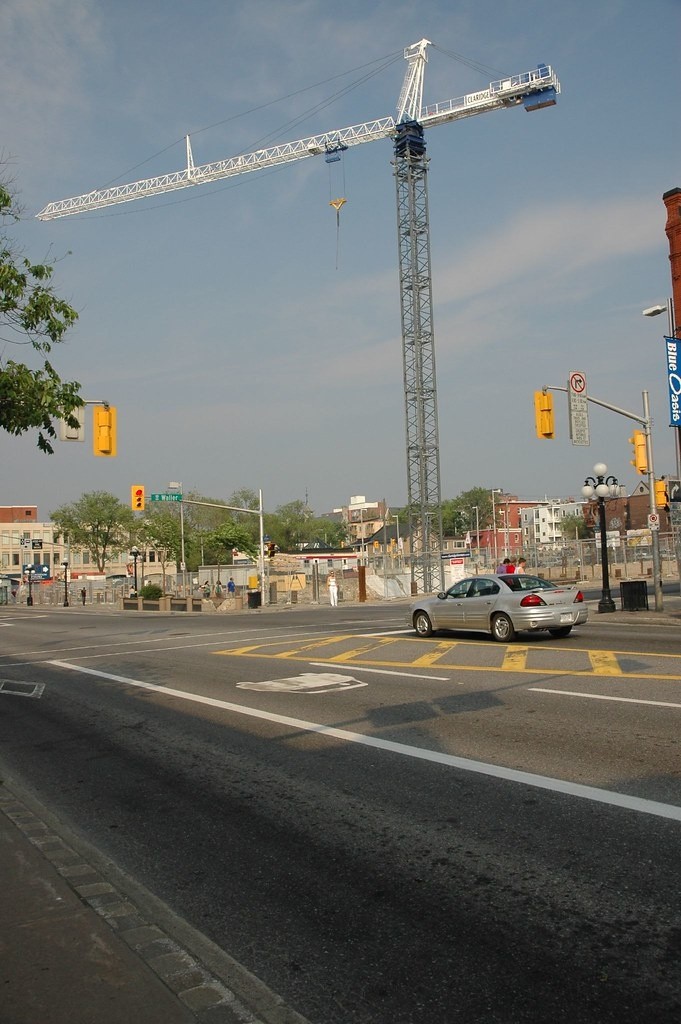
[236, 596, 243, 611]
[619, 580, 651, 611]
[159, 595, 173, 611]
[247, 592, 259, 609]
[254, 592, 261, 606]
[137, 597, 143, 612]
[186, 596, 194, 612]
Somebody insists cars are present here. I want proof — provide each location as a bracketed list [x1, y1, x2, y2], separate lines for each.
[408, 573, 588, 642]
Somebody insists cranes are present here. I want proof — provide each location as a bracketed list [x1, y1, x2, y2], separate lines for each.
[33, 40, 566, 598]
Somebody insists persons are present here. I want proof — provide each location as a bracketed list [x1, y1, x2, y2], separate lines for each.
[214, 581, 223, 596]
[514, 558, 526, 574]
[497, 559, 510, 574]
[507, 560, 516, 585]
[226, 577, 237, 598]
[11, 588, 17, 603]
[130, 585, 134, 594]
[81, 587, 86, 606]
[203, 581, 210, 598]
[327, 571, 338, 606]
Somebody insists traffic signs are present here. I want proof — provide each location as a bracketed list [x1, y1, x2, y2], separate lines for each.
[22, 564, 50, 582]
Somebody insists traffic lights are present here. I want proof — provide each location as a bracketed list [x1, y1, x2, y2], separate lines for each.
[132, 486, 145, 511]
[628, 429, 648, 475]
[268, 543, 275, 558]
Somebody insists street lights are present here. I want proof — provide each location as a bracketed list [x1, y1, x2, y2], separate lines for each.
[23, 563, 35, 606]
[581, 463, 621, 614]
[169, 482, 186, 597]
[492, 489, 501, 559]
[128, 546, 143, 600]
[392, 515, 399, 540]
[59, 558, 69, 607]
[472, 506, 480, 550]
[359, 508, 368, 553]
[642, 297, 680, 480]
